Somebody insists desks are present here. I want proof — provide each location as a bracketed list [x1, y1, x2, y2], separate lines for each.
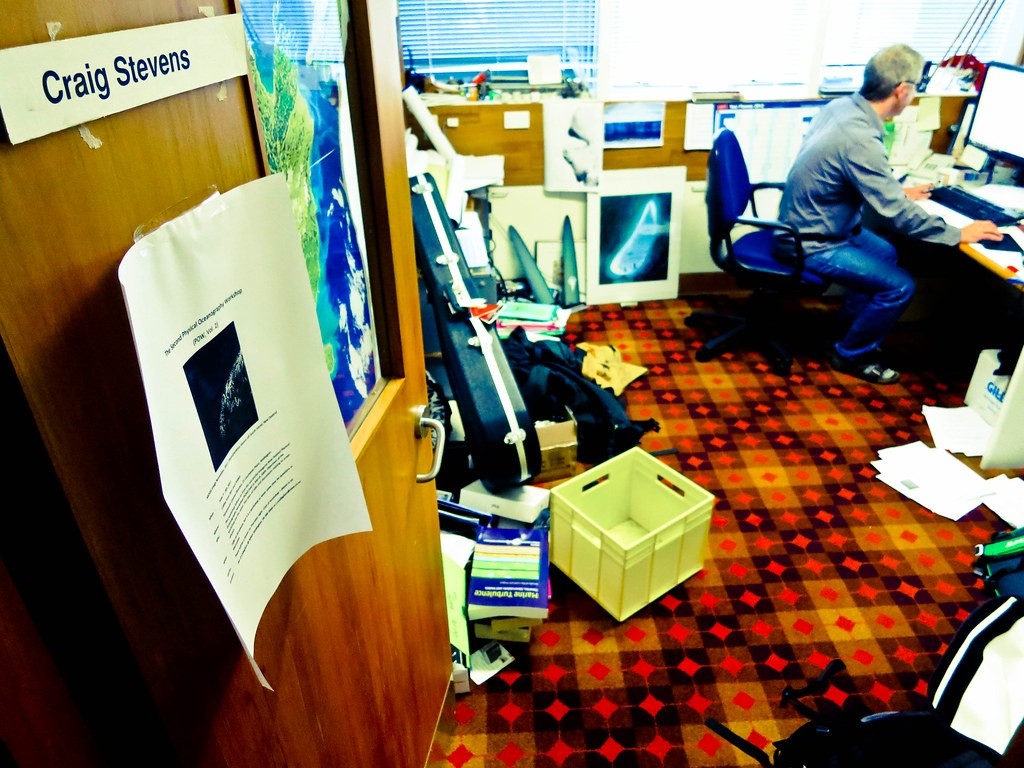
[893, 165, 1024, 292]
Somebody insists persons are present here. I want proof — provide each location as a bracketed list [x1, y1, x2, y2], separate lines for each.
[776, 44, 1003, 382]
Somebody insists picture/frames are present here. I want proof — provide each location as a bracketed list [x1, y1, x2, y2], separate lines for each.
[535, 240, 585, 304]
[585, 165, 687, 305]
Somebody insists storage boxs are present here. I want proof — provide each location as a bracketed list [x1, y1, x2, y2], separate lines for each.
[963, 349, 1009, 427]
[535, 407, 579, 482]
[548, 445, 714, 623]
[409, 173, 542, 494]
[473, 615, 545, 643]
[459, 479, 548, 523]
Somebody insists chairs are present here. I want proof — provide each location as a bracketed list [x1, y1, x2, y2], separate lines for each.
[684, 129, 832, 376]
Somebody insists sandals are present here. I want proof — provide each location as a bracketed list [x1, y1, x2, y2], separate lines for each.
[834, 352, 899, 383]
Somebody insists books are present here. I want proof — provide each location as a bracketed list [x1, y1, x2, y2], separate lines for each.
[465, 527, 549, 643]
[496, 303, 564, 339]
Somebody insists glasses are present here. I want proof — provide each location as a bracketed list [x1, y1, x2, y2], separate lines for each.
[894, 79, 923, 90]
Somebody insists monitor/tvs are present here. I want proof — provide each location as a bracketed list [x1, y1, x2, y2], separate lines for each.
[965, 62, 1024, 187]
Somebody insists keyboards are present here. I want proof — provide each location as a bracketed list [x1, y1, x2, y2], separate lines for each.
[926, 185, 1024, 228]
